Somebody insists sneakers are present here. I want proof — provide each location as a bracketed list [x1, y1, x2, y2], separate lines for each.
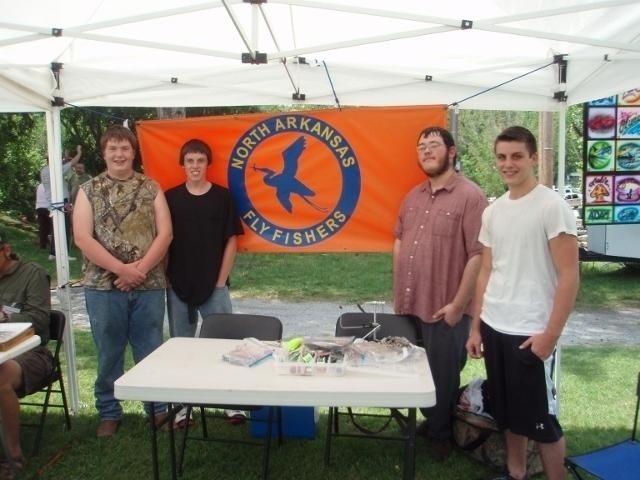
[225, 409, 247, 421]
[175, 409, 194, 427]
[430, 437, 452, 458]
[96, 420, 119, 436]
[155, 415, 178, 431]
[0, 455, 28, 480]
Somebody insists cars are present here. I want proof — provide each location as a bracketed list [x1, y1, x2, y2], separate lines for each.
[552, 183, 583, 210]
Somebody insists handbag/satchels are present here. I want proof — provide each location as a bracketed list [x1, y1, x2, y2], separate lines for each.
[452, 386, 545, 475]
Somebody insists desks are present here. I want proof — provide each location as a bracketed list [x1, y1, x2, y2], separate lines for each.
[0, 334, 41, 366]
[113, 337, 438, 479]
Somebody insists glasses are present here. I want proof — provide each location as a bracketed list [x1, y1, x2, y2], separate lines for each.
[416, 144, 441, 153]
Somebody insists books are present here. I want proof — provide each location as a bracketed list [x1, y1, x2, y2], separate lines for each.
[224, 336, 273, 368]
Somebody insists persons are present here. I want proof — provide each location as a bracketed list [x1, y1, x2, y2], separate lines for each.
[0, 239, 57, 480]
[163, 140, 246, 433]
[392, 126, 488, 463]
[33, 144, 92, 261]
[464, 126, 582, 479]
[72, 125, 173, 440]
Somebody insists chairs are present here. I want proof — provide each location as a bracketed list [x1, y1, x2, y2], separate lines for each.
[324, 312, 423, 479]
[18, 310, 72, 457]
[177, 313, 284, 480]
[563, 372, 640, 480]
